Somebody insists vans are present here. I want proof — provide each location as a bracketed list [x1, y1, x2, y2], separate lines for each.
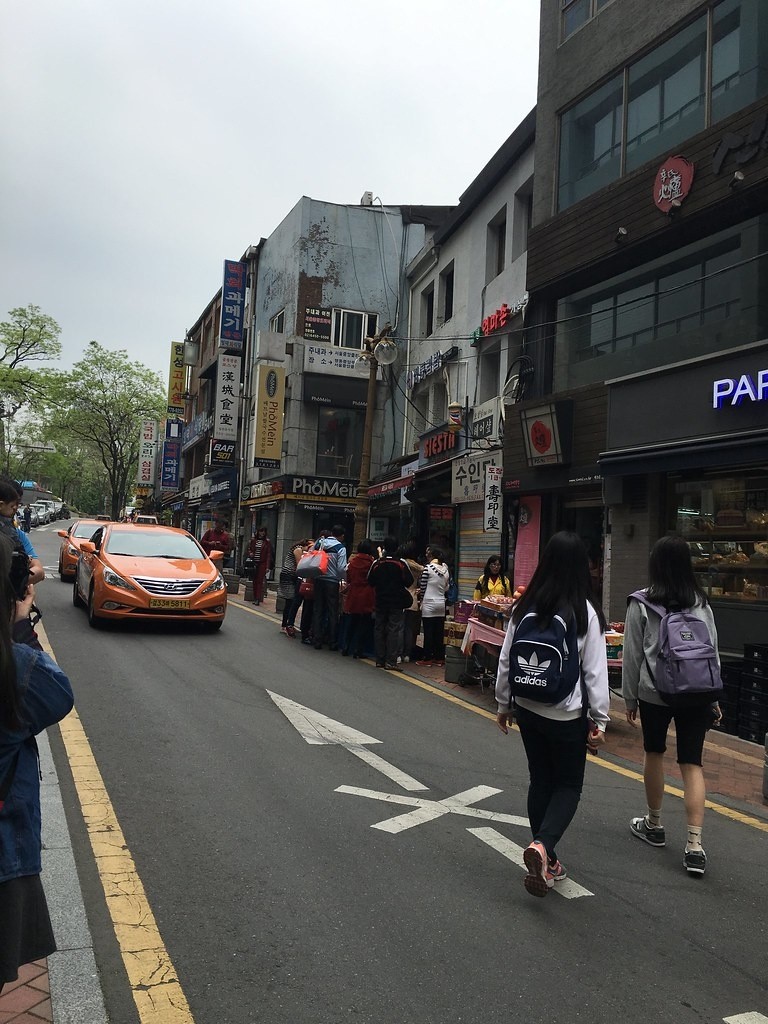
[132, 516, 158, 524]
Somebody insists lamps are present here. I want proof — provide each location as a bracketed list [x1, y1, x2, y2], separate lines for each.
[727, 171, 744, 189]
[666, 199, 682, 220]
[613, 227, 628, 243]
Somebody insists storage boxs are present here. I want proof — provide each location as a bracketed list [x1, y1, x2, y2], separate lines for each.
[710, 716, 740, 736]
[476, 605, 504, 629]
[454, 599, 481, 624]
[738, 642, 768, 745]
[718, 700, 740, 718]
[720, 661, 744, 685]
[718, 683, 739, 703]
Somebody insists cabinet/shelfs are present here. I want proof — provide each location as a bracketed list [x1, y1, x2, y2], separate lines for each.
[665, 527, 768, 606]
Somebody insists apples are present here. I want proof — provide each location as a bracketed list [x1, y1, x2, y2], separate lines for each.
[514, 585, 526, 599]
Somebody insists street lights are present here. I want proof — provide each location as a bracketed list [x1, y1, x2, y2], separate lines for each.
[354, 333, 397, 557]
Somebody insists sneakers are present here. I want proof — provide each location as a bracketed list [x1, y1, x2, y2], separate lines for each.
[630, 815, 668, 847]
[684, 850, 706, 874]
[523, 838, 568, 897]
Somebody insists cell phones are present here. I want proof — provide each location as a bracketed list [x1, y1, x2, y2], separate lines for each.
[587, 716, 598, 756]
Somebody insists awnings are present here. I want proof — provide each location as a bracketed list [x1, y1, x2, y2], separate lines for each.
[367, 452, 467, 500]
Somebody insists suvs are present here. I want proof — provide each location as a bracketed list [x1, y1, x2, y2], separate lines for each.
[35, 500, 56, 521]
[53, 502, 71, 520]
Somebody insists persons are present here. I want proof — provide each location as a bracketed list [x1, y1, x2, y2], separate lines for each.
[0, 476, 73, 999]
[120, 505, 600, 672]
[750, 541, 768, 560]
[621, 536, 723, 874]
[495, 530, 610, 897]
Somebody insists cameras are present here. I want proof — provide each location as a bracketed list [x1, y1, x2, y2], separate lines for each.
[9, 542, 29, 602]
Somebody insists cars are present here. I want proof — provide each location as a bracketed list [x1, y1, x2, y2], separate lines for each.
[14, 510, 26, 531]
[73, 522, 228, 631]
[18, 507, 39, 528]
[30, 504, 50, 525]
[57, 520, 112, 583]
[95, 515, 111, 520]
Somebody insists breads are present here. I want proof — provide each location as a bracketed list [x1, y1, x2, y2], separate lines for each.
[690, 551, 750, 566]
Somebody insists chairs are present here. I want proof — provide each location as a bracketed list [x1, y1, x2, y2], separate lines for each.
[337, 454, 353, 478]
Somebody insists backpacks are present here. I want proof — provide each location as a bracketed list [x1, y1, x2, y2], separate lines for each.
[626, 590, 723, 706]
[508, 605, 581, 707]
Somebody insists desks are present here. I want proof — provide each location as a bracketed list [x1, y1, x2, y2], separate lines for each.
[317, 454, 343, 476]
[461, 617, 506, 674]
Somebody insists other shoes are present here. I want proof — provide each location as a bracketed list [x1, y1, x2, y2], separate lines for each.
[287, 626, 295, 637]
[416, 659, 442, 668]
[302, 638, 313, 645]
[376, 661, 404, 671]
[397, 656, 410, 663]
[280, 626, 287, 633]
[252, 599, 260, 606]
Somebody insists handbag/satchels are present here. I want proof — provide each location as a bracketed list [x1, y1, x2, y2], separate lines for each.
[447, 578, 458, 605]
[296, 550, 328, 578]
[299, 582, 314, 594]
